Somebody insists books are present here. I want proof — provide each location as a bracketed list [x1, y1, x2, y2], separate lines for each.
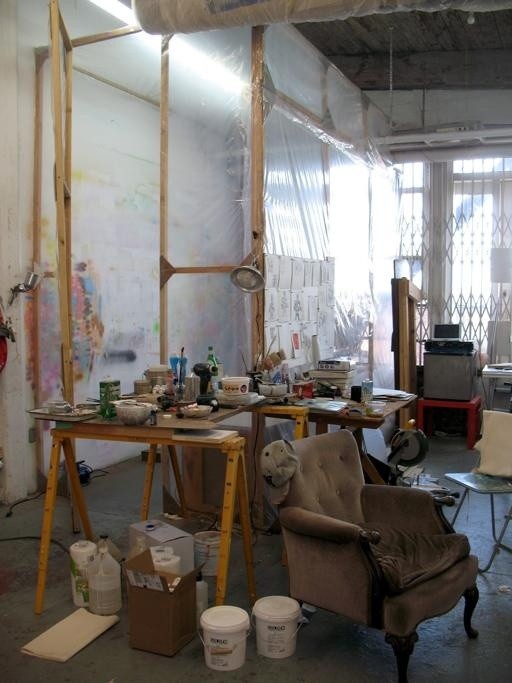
[481, 363, 512, 374]
[488, 362, 512, 368]
[307, 355, 358, 395]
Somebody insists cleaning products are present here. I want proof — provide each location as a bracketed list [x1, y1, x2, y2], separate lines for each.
[205, 346, 220, 376]
[170, 356, 188, 385]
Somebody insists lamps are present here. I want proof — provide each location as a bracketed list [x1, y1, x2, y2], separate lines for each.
[230, 258, 265, 293]
[467, 11, 475, 25]
[8, 271, 38, 306]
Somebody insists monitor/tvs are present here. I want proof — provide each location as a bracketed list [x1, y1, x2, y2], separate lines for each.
[431, 323, 461, 341]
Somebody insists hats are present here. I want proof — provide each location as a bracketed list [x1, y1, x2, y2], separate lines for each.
[260, 439, 299, 505]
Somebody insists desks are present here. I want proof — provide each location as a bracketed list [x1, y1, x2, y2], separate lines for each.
[444, 472, 512, 574]
[417, 394, 482, 450]
[34, 389, 418, 615]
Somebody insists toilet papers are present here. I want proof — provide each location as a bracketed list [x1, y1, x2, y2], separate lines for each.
[152, 554, 181, 574]
[149, 545, 173, 562]
[70, 540, 97, 608]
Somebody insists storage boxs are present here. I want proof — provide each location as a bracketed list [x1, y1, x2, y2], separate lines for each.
[122, 548, 200, 658]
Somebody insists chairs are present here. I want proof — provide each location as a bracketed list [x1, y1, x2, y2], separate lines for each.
[259, 430, 479, 683]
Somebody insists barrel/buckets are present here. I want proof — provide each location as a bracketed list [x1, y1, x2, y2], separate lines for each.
[249, 596, 303, 661]
[87, 545, 123, 615]
[196, 605, 251, 672]
[124, 537, 151, 601]
[195, 570, 209, 630]
[194, 531, 231, 576]
[96, 534, 120, 558]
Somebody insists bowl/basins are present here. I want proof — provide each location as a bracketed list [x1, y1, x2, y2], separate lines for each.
[114, 402, 152, 427]
[257, 383, 287, 397]
[144, 370, 173, 386]
[221, 377, 250, 395]
[134, 380, 153, 395]
[364, 400, 385, 417]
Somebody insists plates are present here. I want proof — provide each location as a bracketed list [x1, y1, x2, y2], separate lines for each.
[180, 404, 212, 417]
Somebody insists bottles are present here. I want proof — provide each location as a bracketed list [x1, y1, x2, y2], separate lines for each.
[169, 344, 189, 381]
[311, 334, 320, 368]
[281, 363, 290, 392]
[195, 569, 210, 630]
[207, 346, 218, 379]
[361, 377, 374, 406]
